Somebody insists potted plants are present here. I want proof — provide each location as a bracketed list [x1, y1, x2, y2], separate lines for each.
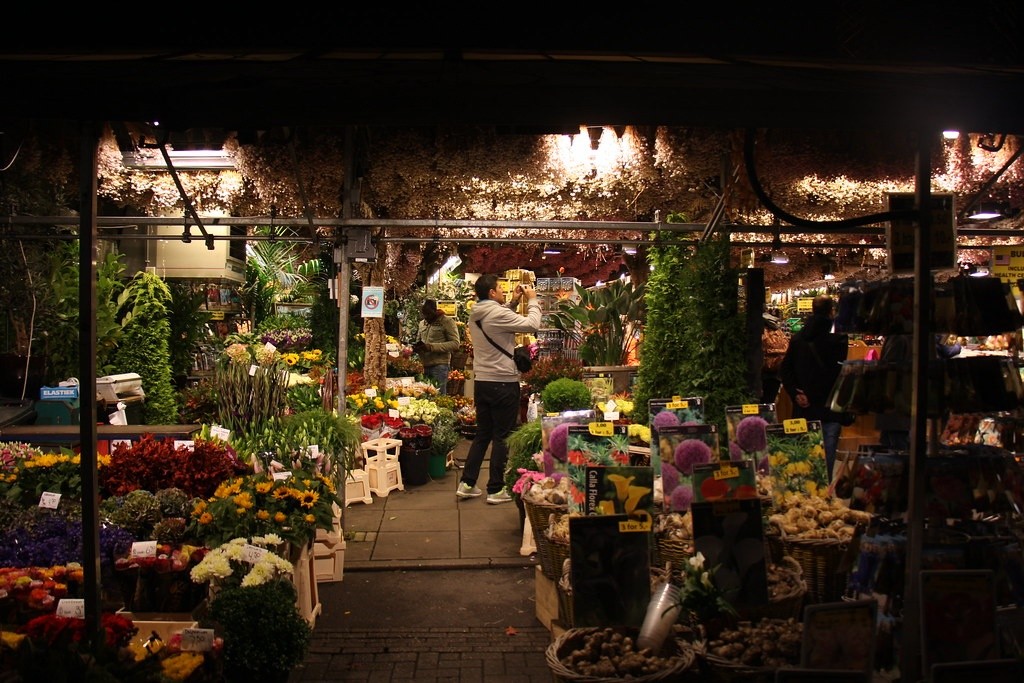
[548, 279, 647, 393]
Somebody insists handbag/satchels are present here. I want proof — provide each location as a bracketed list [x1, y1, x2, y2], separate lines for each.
[513, 347, 532, 373]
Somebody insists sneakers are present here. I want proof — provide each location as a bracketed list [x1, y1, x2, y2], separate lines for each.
[454, 481, 482, 497]
[486, 485, 512, 503]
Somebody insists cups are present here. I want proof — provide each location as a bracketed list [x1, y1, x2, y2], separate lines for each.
[637, 582, 685, 654]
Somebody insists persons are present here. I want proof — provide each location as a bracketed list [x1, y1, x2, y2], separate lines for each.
[779, 296, 848, 482]
[416, 298, 460, 395]
[878, 334, 961, 450]
[456, 275, 542, 504]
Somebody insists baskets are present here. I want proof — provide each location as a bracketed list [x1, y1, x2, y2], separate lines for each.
[520, 478, 1008, 683]
[447, 379, 464, 396]
[451, 352, 468, 371]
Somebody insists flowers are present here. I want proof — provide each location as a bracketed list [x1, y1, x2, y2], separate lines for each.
[0, 321, 471, 683]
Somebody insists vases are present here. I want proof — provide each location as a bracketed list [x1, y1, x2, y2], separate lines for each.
[129, 621, 200, 653]
[289, 540, 321, 630]
[329, 515, 342, 537]
[116, 603, 209, 626]
[398, 444, 432, 485]
[314, 529, 346, 583]
[430, 453, 447, 480]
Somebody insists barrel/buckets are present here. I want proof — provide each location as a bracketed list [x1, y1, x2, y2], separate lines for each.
[400, 448, 433, 485]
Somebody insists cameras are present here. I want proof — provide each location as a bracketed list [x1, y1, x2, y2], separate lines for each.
[520, 285, 529, 292]
